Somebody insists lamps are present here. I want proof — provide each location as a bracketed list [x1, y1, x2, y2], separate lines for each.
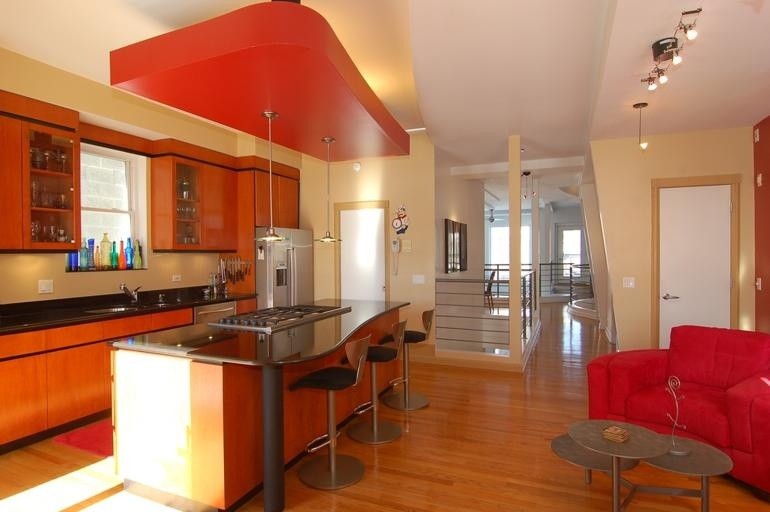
[647, 78, 658, 91]
[671, 51, 682, 65]
[657, 70, 668, 84]
[683, 24, 697, 40]
[252, 109, 286, 243]
[313, 136, 342, 243]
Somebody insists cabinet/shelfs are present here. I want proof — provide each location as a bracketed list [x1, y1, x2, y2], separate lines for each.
[0, 89, 81, 252]
[221, 156, 300, 315]
[151, 137, 237, 253]
[107, 297, 410, 511]
[0, 303, 194, 455]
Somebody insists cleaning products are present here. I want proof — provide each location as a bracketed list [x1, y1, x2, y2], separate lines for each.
[80, 237, 87, 271]
[100, 233, 112, 269]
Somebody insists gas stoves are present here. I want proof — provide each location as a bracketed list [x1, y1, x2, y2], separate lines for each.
[208, 304, 351, 333]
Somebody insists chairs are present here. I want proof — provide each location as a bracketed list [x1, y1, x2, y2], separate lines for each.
[288, 332, 374, 491]
[380, 307, 436, 413]
[339, 318, 407, 446]
[484, 272, 495, 306]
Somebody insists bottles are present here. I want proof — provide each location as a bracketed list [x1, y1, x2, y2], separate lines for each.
[110, 242, 118, 270]
[100, 233, 112, 269]
[119, 241, 126, 269]
[125, 238, 133, 268]
[80, 243, 88, 271]
[134, 241, 142, 269]
[68, 249, 78, 272]
[88, 239, 96, 270]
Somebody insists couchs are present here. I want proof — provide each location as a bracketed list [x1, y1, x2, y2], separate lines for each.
[584, 324, 770, 495]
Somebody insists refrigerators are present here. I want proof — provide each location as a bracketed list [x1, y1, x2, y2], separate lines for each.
[256, 323, 315, 365]
[255, 227, 314, 309]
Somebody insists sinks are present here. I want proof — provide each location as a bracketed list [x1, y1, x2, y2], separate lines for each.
[84, 307, 137, 314]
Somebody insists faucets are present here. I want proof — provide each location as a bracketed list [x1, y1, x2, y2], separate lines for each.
[120, 283, 141, 306]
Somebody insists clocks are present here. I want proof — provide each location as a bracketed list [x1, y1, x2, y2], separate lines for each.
[392, 218, 402, 229]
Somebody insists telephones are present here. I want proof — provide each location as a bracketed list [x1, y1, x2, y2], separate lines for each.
[392, 238, 400, 274]
